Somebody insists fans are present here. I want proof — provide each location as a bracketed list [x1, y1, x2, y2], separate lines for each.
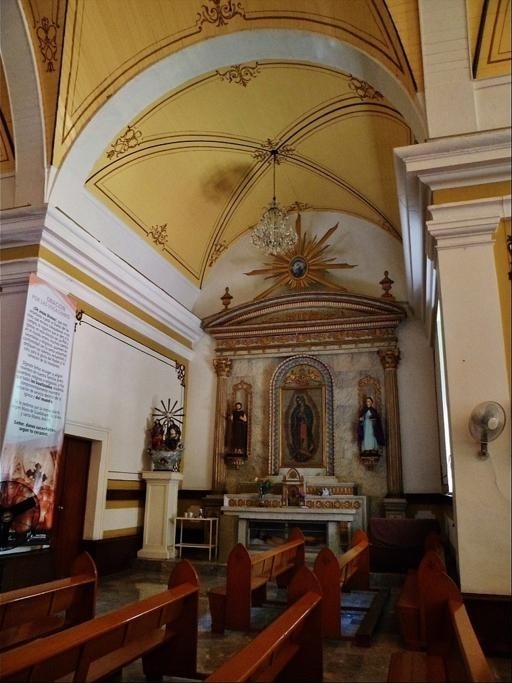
[468, 400, 506, 456]
[0, 480, 41, 550]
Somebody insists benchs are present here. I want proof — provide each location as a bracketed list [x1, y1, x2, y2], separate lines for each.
[0, 528, 497, 682]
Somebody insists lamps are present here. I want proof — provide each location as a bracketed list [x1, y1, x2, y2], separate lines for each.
[249, 150, 297, 255]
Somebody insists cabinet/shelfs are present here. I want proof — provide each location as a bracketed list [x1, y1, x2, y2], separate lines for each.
[172, 517, 219, 563]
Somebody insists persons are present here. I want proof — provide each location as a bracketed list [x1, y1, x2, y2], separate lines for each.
[359, 397, 382, 457]
[291, 395, 313, 461]
[230, 402, 247, 453]
